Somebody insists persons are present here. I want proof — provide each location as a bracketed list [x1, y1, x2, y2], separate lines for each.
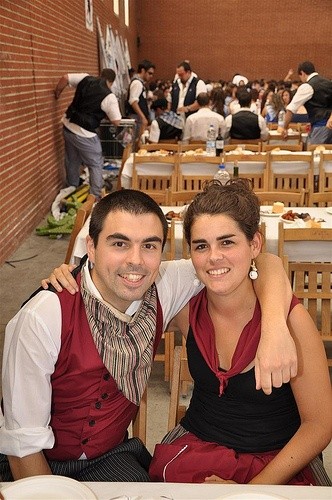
[57, 58, 332, 203]
[0, 189, 299, 482]
[41, 177, 332, 487]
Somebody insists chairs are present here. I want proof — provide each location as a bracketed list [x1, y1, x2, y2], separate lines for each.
[59, 119, 332, 486]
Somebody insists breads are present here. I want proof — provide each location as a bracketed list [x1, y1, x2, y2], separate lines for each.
[272, 201, 284, 213]
[138, 148, 204, 155]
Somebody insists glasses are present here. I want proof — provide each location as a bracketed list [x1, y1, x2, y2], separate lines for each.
[144, 70, 152, 75]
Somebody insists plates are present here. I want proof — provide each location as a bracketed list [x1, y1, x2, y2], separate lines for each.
[259, 208, 287, 217]
[0, 475, 96, 500]
[165, 217, 184, 223]
[279, 213, 323, 222]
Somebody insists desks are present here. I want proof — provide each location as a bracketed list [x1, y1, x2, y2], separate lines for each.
[67, 205, 332, 270]
[139, 127, 308, 146]
[118, 151, 332, 188]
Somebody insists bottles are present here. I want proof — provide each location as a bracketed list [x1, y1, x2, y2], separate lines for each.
[255, 99, 261, 115]
[215, 127, 224, 157]
[278, 108, 286, 133]
[214, 165, 230, 186]
[206, 125, 215, 154]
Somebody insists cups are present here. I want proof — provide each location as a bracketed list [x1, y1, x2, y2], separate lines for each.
[178, 141, 187, 156]
